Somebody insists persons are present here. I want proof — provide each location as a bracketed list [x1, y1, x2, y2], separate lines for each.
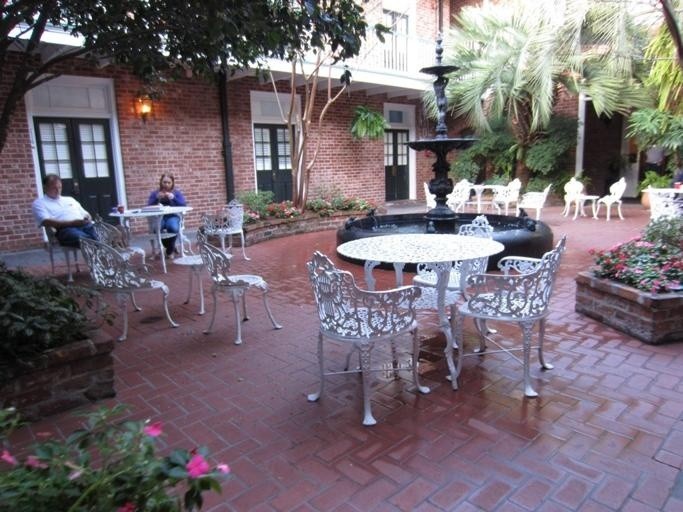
[148, 174, 187, 260]
[641, 147, 667, 178]
[32, 174, 107, 248]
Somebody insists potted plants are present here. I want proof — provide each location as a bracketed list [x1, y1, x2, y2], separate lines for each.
[636, 171, 671, 209]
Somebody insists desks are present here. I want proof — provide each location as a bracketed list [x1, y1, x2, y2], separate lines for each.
[466, 185, 503, 215]
[109, 207, 193, 274]
[336, 233, 505, 390]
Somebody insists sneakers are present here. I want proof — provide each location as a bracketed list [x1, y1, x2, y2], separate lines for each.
[165, 244, 179, 259]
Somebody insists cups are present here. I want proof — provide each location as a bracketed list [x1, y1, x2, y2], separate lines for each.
[118, 207, 124, 214]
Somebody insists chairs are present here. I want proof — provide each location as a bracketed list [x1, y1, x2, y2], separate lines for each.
[516, 183, 552, 220]
[446, 235, 566, 397]
[592, 177, 627, 221]
[424, 182, 436, 211]
[448, 179, 470, 213]
[563, 177, 587, 217]
[196, 231, 282, 345]
[79, 237, 180, 341]
[305, 251, 431, 426]
[413, 215, 497, 350]
[41, 221, 95, 282]
[647, 185, 683, 225]
[201, 199, 251, 260]
[493, 178, 522, 216]
[146, 213, 194, 260]
[93, 213, 147, 271]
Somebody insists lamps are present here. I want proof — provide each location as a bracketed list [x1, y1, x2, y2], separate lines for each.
[132, 90, 152, 124]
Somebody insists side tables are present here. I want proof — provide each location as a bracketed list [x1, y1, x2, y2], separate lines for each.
[173, 253, 232, 315]
[573, 195, 600, 221]
